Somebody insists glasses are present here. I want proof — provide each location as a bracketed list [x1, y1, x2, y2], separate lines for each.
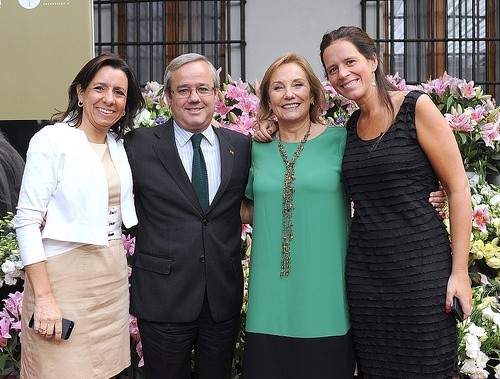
[169, 86, 214, 99]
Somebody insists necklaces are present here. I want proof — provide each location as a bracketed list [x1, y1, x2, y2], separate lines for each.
[278, 121, 313, 278]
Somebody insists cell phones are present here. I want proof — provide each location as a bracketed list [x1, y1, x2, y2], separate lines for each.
[29, 313, 75, 340]
[452, 297, 463, 321]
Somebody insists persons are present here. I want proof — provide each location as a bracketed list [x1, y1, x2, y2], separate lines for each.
[12, 52, 146, 379]
[40, 52, 252, 379]
[239, 53, 447, 379]
[253, 27, 474, 379]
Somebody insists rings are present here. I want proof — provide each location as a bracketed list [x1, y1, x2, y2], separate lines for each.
[39, 328, 46, 333]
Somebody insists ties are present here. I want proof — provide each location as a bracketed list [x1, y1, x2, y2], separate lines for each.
[189, 132, 209, 215]
